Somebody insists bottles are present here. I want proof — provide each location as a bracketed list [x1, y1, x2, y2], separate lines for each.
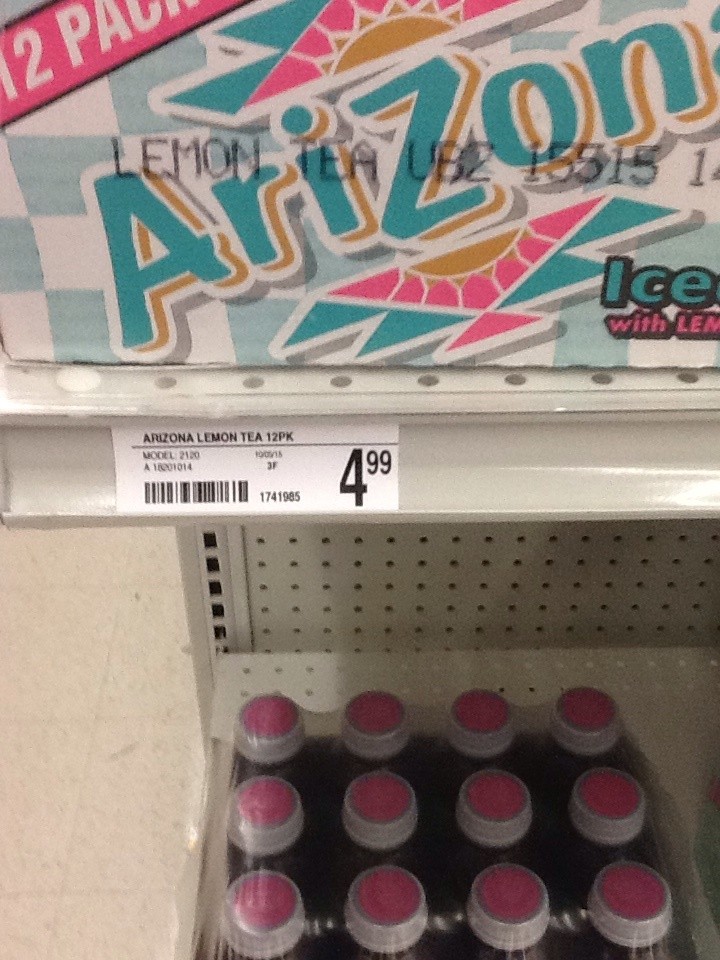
[218, 686, 685, 960]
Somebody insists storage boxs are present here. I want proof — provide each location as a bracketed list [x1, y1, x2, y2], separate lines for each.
[0, 0, 720, 373]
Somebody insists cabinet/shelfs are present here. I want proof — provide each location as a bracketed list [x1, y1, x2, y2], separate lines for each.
[1, 360, 718, 960]
